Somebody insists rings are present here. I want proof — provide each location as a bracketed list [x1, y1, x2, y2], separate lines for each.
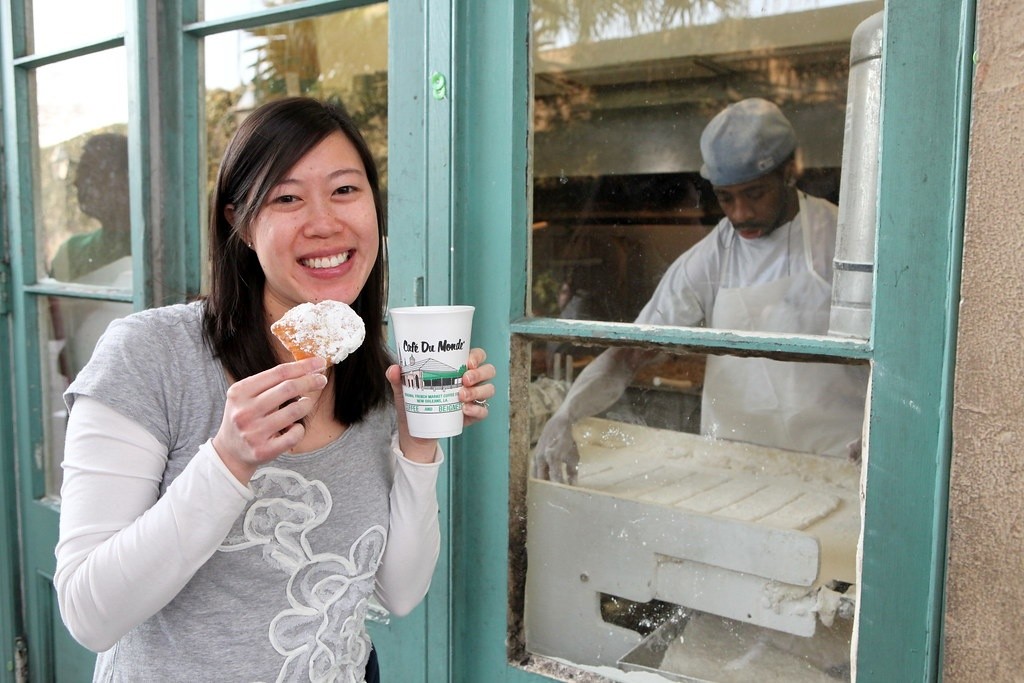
[474, 398, 486, 406]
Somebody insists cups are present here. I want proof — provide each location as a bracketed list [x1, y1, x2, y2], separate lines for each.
[390, 306, 476, 438]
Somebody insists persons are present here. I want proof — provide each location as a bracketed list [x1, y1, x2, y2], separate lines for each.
[532, 97, 871, 489]
[532, 245, 625, 380]
[42, 132, 132, 341]
[51, 99, 495, 682]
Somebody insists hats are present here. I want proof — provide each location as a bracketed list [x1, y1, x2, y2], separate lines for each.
[700, 97, 798, 184]
